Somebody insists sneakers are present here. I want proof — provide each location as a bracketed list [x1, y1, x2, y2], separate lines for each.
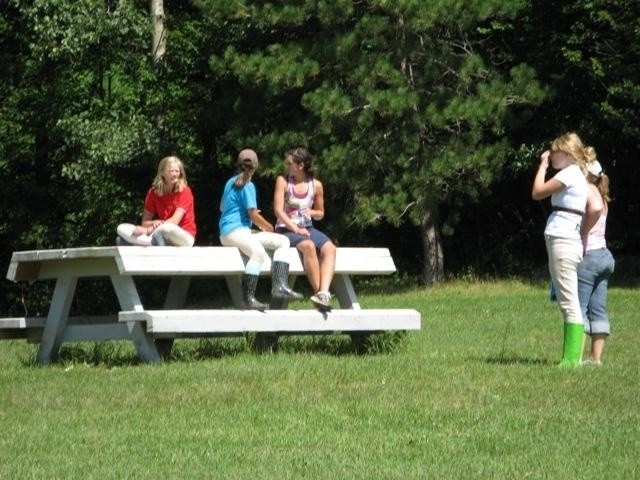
[310, 292, 331, 312]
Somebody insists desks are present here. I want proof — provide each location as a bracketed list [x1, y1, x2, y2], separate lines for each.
[6, 243, 396, 363]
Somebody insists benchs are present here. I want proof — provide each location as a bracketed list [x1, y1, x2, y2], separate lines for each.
[0, 315, 118, 340]
[118, 309, 421, 335]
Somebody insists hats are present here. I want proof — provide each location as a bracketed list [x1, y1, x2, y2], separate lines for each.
[586, 157, 604, 179]
[238, 149, 259, 165]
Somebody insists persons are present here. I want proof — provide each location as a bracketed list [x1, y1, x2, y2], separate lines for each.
[114, 155, 198, 248]
[273, 148, 339, 312]
[549, 159, 618, 365]
[217, 148, 304, 312]
[530, 132, 597, 370]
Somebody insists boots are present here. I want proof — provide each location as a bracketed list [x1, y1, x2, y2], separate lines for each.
[553, 322, 584, 371]
[240, 273, 267, 310]
[271, 261, 304, 301]
